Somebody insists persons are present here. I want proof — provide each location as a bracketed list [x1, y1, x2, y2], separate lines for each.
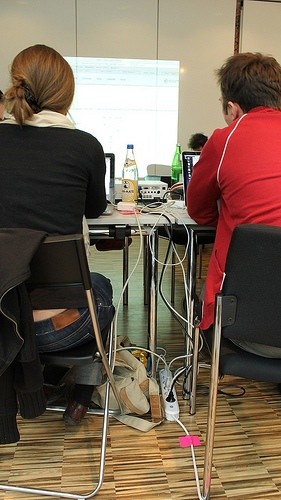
[186, 52, 281, 359]
[187, 134, 208, 151]
[0, 44, 108, 426]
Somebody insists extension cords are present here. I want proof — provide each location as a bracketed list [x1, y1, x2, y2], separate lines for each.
[159, 369, 180, 422]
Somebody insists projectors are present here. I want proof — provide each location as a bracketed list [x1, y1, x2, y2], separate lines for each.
[113, 176, 169, 201]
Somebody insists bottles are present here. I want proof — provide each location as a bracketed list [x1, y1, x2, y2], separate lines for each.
[171, 145, 183, 194]
[121, 145, 138, 202]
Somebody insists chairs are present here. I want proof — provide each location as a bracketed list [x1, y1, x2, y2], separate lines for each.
[0, 226, 126, 500]
[194, 224, 281, 500]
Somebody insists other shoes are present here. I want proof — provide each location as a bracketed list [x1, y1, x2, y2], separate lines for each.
[62, 400, 88, 426]
[187, 347, 212, 362]
[43, 385, 65, 406]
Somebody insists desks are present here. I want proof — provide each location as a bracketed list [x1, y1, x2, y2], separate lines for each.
[86, 198, 217, 401]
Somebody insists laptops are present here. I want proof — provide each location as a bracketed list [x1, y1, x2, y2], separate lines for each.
[102, 153, 117, 215]
[180, 150, 203, 209]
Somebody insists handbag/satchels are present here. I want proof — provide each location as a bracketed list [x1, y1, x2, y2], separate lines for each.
[93, 335, 164, 432]
